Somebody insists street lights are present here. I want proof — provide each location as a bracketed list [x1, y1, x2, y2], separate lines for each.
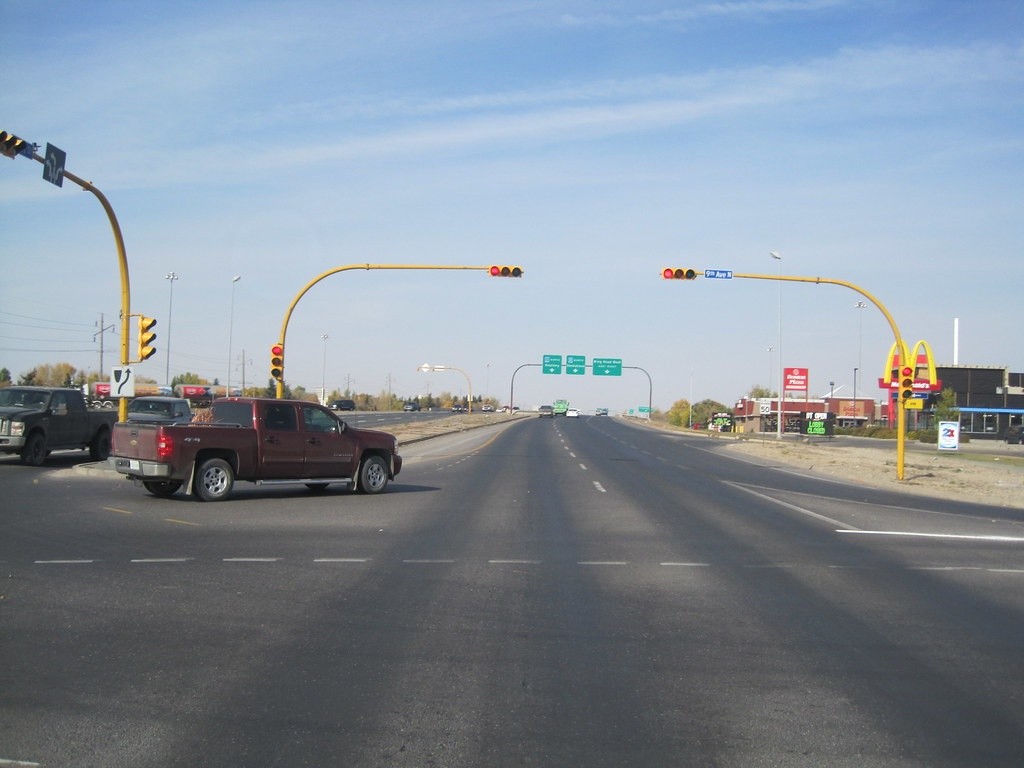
[166, 271, 179, 385]
[830, 382, 834, 398]
[226, 276, 241, 396]
[770, 250, 783, 439]
[854, 368, 858, 428]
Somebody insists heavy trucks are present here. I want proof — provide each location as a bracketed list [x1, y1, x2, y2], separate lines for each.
[69, 382, 242, 410]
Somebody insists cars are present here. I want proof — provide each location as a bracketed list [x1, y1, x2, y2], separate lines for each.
[403, 403, 519, 413]
[1004, 425, 1024, 445]
[539, 399, 608, 418]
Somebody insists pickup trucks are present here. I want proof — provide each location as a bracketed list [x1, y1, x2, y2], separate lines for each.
[0, 386, 196, 466]
[111, 396, 403, 502]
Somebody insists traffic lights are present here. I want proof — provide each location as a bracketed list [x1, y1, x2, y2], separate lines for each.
[0, 130, 27, 160]
[138, 316, 156, 360]
[899, 366, 915, 400]
[271, 345, 283, 379]
[662, 268, 697, 280]
[489, 265, 523, 278]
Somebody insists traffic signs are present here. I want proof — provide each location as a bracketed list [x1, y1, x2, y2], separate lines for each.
[592, 358, 622, 376]
[566, 355, 585, 375]
[542, 354, 562, 374]
